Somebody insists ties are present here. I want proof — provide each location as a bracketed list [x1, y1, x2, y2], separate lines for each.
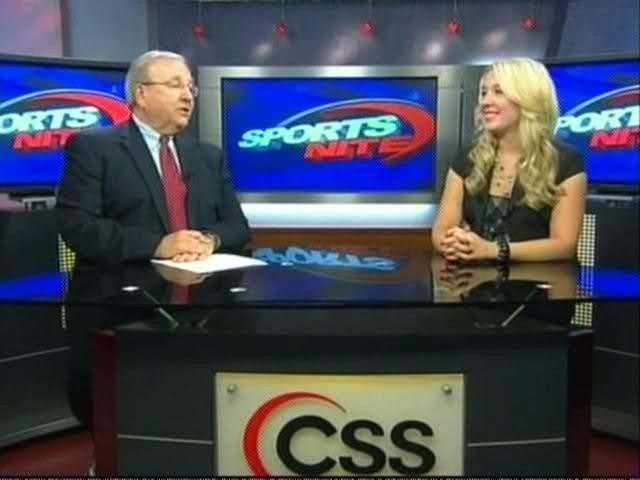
[160, 135, 192, 234]
[169, 282, 189, 305]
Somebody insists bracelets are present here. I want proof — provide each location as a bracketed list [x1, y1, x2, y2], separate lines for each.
[496, 234, 512, 262]
[200, 231, 220, 251]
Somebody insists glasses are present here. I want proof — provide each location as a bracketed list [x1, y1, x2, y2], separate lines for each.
[141, 78, 199, 98]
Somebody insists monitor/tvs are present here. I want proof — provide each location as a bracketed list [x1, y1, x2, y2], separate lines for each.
[1, 50, 196, 205]
[468, 51, 639, 194]
[196, 64, 463, 203]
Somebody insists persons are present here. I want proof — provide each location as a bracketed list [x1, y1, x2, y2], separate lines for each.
[54, 48, 254, 476]
[430, 57, 589, 327]
[72, 253, 238, 307]
[431, 263, 574, 300]
